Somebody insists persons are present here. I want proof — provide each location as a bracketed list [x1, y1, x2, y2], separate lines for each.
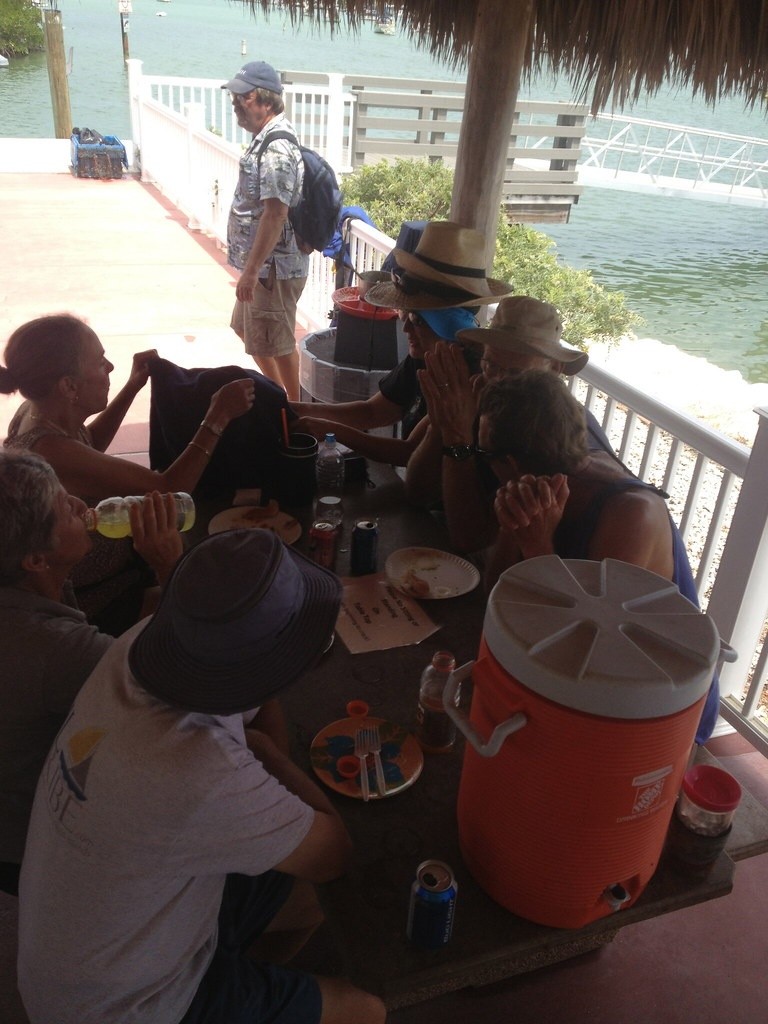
[478, 371, 720, 764]
[287, 221, 512, 533]
[1, 314, 255, 638]
[17, 526, 388, 1024]
[220, 60, 309, 402]
[405, 296, 615, 553]
[0, 448, 186, 896]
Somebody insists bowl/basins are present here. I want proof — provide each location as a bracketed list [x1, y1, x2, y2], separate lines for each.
[675, 761, 743, 837]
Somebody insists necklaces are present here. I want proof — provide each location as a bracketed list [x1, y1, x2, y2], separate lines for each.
[29, 410, 84, 439]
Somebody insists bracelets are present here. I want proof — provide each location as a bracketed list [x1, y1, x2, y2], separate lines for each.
[189, 442, 211, 459]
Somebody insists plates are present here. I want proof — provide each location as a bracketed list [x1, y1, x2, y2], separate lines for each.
[385, 548, 480, 600]
[309, 715, 424, 800]
[207, 506, 302, 548]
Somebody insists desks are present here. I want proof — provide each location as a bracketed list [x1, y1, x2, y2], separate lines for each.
[182, 453, 737, 1014]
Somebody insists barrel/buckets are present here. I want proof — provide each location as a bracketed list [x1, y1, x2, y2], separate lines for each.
[443, 554, 740, 930]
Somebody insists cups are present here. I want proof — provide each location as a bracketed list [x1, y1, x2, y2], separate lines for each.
[276, 433, 318, 508]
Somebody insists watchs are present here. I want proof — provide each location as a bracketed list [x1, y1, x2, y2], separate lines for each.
[442, 439, 474, 460]
[201, 420, 224, 438]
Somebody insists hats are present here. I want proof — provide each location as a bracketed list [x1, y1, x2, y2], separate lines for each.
[457, 296, 589, 376]
[221, 61, 284, 95]
[364, 269, 513, 310]
[129, 528, 343, 717]
[394, 222, 514, 296]
[419, 308, 480, 342]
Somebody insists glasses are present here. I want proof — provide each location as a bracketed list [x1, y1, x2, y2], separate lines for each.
[391, 268, 424, 295]
[475, 444, 508, 464]
[399, 309, 424, 326]
[227, 92, 253, 101]
[479, 358, 527, 379]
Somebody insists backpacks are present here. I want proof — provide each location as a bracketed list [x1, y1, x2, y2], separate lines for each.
[257, 130, 343, 252]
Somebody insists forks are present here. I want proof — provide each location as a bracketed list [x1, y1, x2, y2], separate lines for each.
[352, 729, 371, 801]
[366, 726, 386, 797]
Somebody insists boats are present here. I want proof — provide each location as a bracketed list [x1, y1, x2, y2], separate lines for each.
[374, 15, 394, 35]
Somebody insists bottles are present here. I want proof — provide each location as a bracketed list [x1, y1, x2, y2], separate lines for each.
[83, 489, 195, 537]
[316, 432, 345, 527]
[417, 653, 463, 755]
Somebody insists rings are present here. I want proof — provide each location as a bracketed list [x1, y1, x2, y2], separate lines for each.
[438, 383, 450, 393]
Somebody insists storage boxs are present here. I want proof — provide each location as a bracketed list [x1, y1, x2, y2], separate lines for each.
[70, 134, 128, 180]
